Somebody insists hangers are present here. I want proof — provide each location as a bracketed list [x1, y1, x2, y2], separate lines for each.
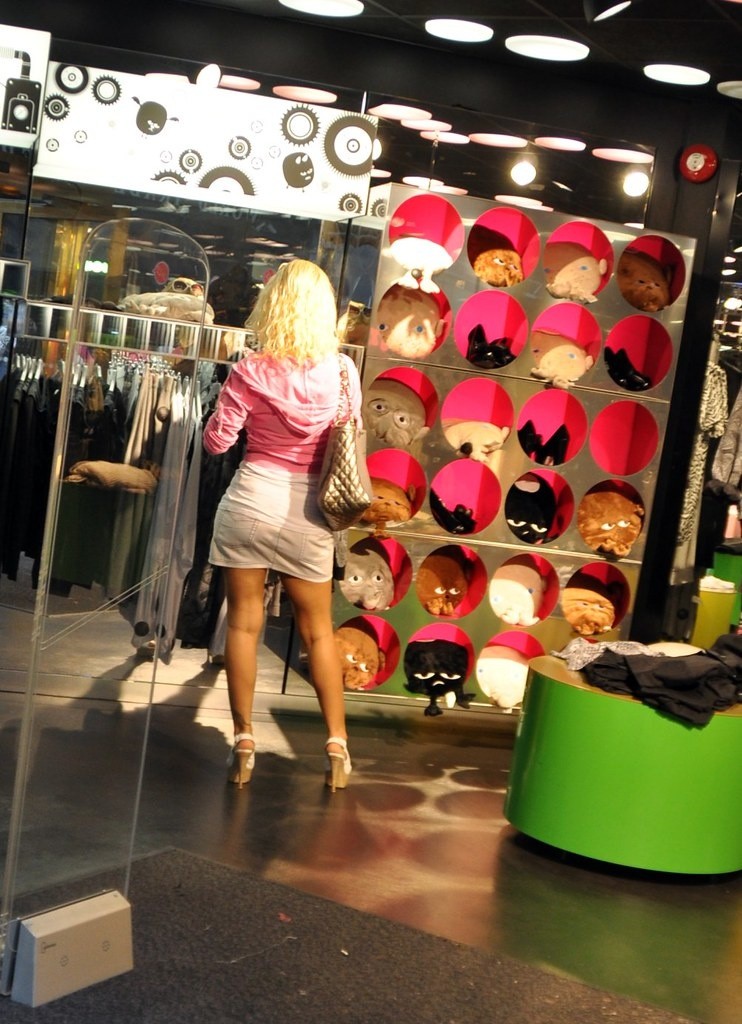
[15, 353, 222, 423]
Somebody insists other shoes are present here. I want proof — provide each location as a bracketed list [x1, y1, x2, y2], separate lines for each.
[148, 640, 175, 652]
[429, 489, 476, 534]
[604, 346, 651, 391]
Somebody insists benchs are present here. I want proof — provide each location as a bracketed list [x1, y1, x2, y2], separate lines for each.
[504, 655, 742, 875]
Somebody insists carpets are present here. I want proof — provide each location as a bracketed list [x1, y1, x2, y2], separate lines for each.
[0, 847, 695, 1024]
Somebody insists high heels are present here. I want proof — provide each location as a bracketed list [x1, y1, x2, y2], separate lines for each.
[466, 323, 516, 369]
[517, 419, 569, 465]
[226, 733, 256, 790]
[325, 736, 352, 792]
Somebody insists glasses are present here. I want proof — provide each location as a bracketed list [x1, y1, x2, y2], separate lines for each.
[173, 279, 204, 296]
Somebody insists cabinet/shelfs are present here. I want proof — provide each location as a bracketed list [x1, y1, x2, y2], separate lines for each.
[287, 182, 700, 718]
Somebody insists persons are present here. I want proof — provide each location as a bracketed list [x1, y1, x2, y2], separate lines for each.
[203, 259, 365, 794]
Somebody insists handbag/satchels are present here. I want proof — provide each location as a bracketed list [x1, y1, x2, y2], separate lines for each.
[317, 354, 371, 531]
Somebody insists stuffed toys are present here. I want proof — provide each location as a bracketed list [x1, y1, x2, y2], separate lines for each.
[331, 233, 678, 717]
